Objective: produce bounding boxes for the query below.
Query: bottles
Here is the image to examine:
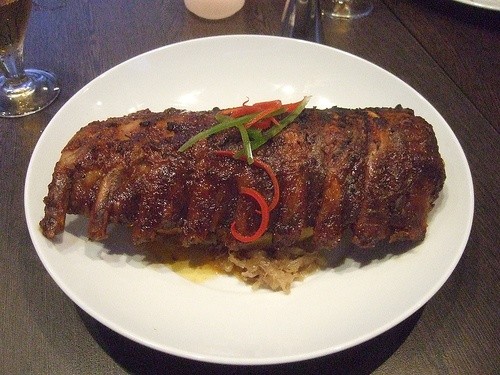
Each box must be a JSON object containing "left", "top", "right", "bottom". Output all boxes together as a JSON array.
[{"left": 280, "top": 0, "right": 322, "bottom": 45}]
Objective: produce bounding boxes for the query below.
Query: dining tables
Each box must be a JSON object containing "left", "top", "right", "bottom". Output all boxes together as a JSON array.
[{"left": 0, "top": 0, "right": 500, "bottom": 375}]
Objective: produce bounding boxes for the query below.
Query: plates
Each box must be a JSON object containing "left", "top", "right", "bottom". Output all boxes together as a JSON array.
[{"left": 23, "top": 34, "right": 475, "bottom": 366}]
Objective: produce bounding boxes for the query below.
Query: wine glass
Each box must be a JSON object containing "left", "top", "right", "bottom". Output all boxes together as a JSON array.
[
  {"left": 321, "top": 0, "right": 375, "bottom": 19},
  {"left": 0, "top": 0, "right": 61, "bottom": 117}
]
